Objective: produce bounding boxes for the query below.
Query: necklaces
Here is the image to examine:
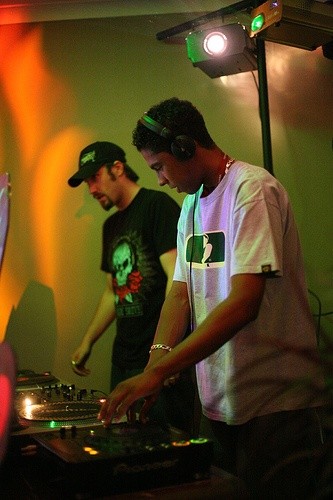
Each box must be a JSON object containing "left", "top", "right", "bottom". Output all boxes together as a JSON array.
[{"left": 219, "top": 153, "right": 234, "bottom": 183}]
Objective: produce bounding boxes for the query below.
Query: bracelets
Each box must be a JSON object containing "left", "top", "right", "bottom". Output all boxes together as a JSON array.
[{"left": 149, "top": 344, "right": 173, "bottom": 354}]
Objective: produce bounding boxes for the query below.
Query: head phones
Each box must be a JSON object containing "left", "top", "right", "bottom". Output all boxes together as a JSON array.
[{"left": 139, "top": 112, "right": 196, "bottom": 161}]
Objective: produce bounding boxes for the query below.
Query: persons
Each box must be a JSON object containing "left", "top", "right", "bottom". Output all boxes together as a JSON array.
[
  {"left": 97, "top": 97, "right": 328, "bottom": 477},
  {"left": 68, "top": 141, "right": 196, "bottom": 437}
]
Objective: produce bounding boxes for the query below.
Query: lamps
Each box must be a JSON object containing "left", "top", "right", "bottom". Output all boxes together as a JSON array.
[{"left": 185, "top": 23, "right": 258, "bottom": 79}]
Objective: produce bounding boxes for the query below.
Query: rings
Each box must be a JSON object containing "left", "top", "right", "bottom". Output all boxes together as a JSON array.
[{"left": 71, "top": 360, "right": 76, "bottom": 365}]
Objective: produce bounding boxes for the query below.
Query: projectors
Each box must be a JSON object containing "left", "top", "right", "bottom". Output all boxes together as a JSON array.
[{"left": 185, "top": 23, "right": 259, "bottom": 79}]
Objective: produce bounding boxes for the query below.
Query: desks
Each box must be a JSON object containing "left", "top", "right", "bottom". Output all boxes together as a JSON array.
[{"left": 21, "top": 464, "right": 243, "bottom": 500}]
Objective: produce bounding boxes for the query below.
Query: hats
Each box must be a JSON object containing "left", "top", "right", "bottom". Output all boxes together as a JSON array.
[{"left": 68, "top": 141, "right": 126, "bottom": 188}]
